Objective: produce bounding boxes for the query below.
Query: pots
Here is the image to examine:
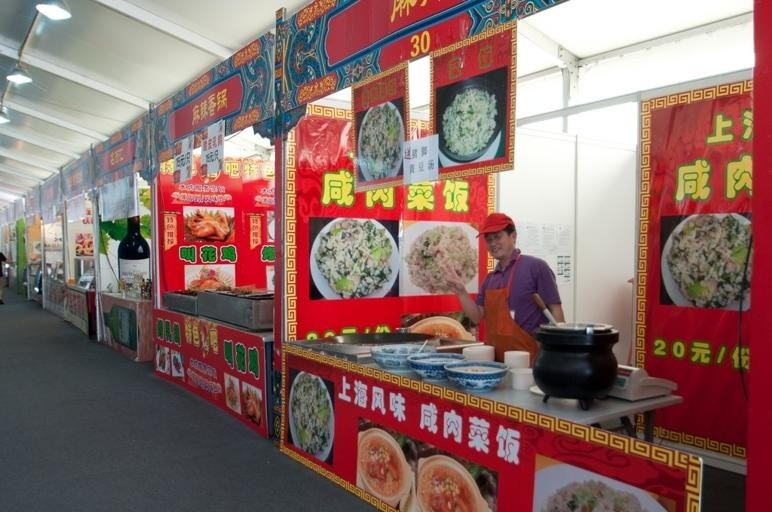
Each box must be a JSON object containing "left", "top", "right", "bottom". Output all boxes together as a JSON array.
[
  {"left": 533, "top": 323, "right": 618, "bottom": 410},
  {"left": 357, "top": 428, "right": 414, "bottom": 503},
  {"left": 413, "top": 454, "right": 489, "bottom": 512},
  {"left": 438, "top": 78, "right": 503, "bottom": 160}
]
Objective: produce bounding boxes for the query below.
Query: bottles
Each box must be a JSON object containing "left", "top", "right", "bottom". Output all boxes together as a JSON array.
[{"left": 115, "top": 216, "right": 149, "bottom": 293}]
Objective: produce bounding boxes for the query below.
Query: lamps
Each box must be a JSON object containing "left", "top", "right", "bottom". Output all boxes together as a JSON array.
[
  {"left": 35, "top": 1, "right": 72, "bottom": 20},
  {"left": 6, "top": 60, "right": 32, "bottom": 83},
  {"left": 0, "top": 108, "right": 9, "bottom": 120}
]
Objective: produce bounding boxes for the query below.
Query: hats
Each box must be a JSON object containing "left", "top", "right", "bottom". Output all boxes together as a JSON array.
[{"left": 475, "top": 215, "right": 517, "bottom": 236}]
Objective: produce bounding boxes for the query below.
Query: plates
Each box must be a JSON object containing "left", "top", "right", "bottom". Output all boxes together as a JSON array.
[
  {"left": 156, "top": 350, "right": 170, "bottom": 373},
  {"left": 357, "top": 102, "right": 405, "bottom": 181},
  {"left": 533, "top": 463, "right": 669, "bottom": 511},
  {"left": 242, "top": 381, "right": 261, "bottom": 426},
  {"left": 172, "top": 353, "right": 186, "bottom": 379},
  {"left": 224, "top": 373, "right": 241, "bottom": 415}
]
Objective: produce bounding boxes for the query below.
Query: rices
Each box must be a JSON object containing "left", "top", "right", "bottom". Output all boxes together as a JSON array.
[
  {"left": 361, "top": 104, "right": 402, "bottom": 178},
  {"left": 441, "top": 86, "right": 499, "bottom": 156},
  {"left": 542, "top": 475, "right": 645, "bottom": 512}
]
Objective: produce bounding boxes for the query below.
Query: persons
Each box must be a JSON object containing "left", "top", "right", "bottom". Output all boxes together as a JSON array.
[
  {"left": 1, "top": 251, "right": 8, "bottom": 303},
  {"left": 446, "top": 214, "right": 565, "bottom": 368}
]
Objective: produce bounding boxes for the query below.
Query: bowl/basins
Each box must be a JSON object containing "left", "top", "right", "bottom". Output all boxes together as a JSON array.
[
  {"left": 444, "top": 359, "right": 508, "bottom": 393},
  {"left": 370, "top": 343, "right": 436, "bottom": 374},
  {"left": 407, "top": 316, "right": 473, "bottom": 342},
  {"left": 408, "top": 353, "right": 464, "bottom": 383}
]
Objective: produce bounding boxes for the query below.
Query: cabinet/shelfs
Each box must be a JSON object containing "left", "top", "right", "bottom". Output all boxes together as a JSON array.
[
  {"left": 159, "top": 307, "right": 274, "bottom": 439},
  {"left": 68, "top": 284, "right": 96, "bottom": 339},
  {"left": 45, "top": 277, "right": 65, "bottom": 318},
  {"left": 285, "top": 343, "right": 682, "bottom": 512},
  {"left": 100, "top": 293, "right": 153, "bottom": 362}
]
